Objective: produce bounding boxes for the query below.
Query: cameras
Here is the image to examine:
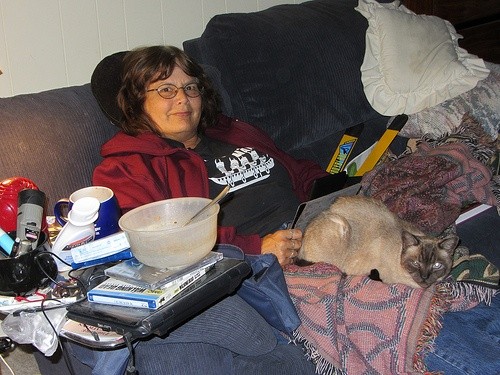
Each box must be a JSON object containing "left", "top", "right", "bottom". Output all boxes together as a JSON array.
[{"left": 0, "top": 254, "right": 57, "bottom": 296}]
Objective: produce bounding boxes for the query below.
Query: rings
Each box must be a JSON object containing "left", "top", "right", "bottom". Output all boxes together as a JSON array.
[
  {"left": 288, "top": 229, "right": 293, "bottom": 240},
  {"left": 288, "top": 249, "right": 294, "bottom": 259},
  {"left": 290, "top": 240, "right": 295, "bottom": 249}
]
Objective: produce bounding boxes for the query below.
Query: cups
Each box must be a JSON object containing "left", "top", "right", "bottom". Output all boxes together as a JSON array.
[
  {"left": 0, "top": 229, "right": 58, "bottom": 297},
  {"left": 54, "top": 186, "right": 123, "bottom": 241}
]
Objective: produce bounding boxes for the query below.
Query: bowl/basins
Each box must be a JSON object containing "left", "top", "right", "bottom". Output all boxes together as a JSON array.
[{"left": 120, "top": 194, "right": 221, "bottom": 269}]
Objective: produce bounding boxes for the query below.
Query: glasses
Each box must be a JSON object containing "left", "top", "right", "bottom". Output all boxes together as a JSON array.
[{"left": 144, "top": 82, "right": 204, "bottom": 99}]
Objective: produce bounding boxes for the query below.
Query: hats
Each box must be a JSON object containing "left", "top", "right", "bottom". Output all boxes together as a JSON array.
[{"left": 91, "top": 51, "right": 139, "bottom": 127}]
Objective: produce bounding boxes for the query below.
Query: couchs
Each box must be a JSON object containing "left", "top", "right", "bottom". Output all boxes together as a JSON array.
[{"left": 0, "top": 0, "right": 500, "bottom": 375}]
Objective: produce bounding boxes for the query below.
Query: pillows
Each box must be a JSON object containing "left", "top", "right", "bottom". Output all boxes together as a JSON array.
[{"left": 351, "top": 0, "right": 490, "bottom": 116}]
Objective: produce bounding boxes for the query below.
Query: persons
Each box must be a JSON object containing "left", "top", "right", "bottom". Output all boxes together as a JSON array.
[{"left": 93, "top": 44, "right": 500, "bottom": 375}]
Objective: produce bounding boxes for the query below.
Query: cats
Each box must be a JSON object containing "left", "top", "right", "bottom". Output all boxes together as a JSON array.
[{"left": 290, "top": 194, "right": 460, "bottom": 290}]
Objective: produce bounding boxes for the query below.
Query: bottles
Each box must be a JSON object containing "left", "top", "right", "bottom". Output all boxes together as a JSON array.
[{"left": 50, "top": 197, "right": 99, "bottom": 272}]
